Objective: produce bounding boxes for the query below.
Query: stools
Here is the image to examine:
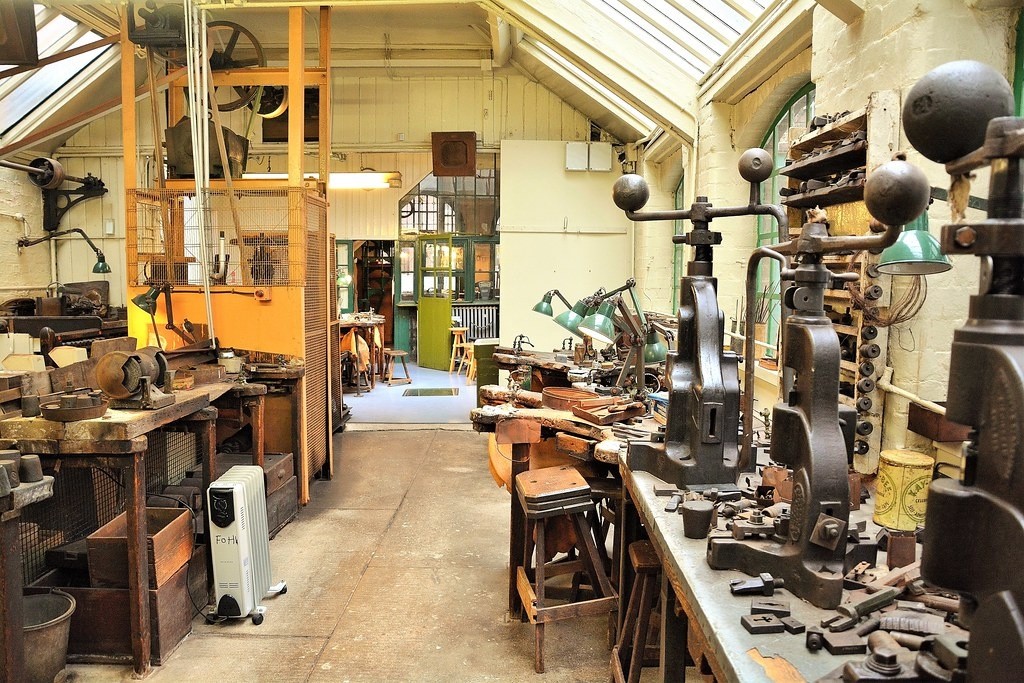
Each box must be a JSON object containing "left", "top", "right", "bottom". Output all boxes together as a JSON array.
[
  {"left": 516, "top": 465, "right": 619, "bottom": 673},
  {"left": 607, "top": 541, "right": 660, "bottom": 683},
  {"left": 449, "top": 327, "right": 477, "bottom": 386},
  {"left": 341, "top": 348, "right": 412, "bottom": 393}
]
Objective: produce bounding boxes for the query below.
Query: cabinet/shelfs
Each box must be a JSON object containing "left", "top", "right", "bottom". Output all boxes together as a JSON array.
[{"left": 362, "top": 241, "right": 395, "bottom": 345}]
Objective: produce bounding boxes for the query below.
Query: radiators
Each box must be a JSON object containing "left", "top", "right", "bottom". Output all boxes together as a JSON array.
[
  {"left": 452, "top": 307, "right": 498, "bottom": 342},
  {"left": 205, "top": 465, "right": 288, "bottom": 623}
]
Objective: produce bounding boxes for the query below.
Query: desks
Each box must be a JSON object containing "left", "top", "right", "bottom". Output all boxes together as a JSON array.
[
  {"left": 339, "top": 315, "right": 387, "bottom": 387},
  {"left": 618, "top": 444, "right": 921, "bottom": 683}
]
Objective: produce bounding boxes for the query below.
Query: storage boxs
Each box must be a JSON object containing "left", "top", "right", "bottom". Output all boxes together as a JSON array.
[{"left": 26, "top": 452, "right": 300, "bottom": 665}]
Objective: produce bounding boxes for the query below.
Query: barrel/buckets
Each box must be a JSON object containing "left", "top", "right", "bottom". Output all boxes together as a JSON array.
[{"left": 22, "top": 587, "right": 76, "bottom": 683}]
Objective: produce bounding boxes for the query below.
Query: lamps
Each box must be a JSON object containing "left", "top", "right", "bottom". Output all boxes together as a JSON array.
[
  {"left": 531, "top": 278, "right": 670, "bottom": 393},
  {"left": 241, "top": 172, "right": 402, "bottom": 187},
  {"left": 336, "top": 268, "right": 352, "bottom": 286},
  {"left": 19, "top": 230, "right": 112, "bottom": 273},
  {"left": 876, "top": 187, "right": 988, "bottom": 276},
  {"left": 130, "top": 281, "right": 193, "bottom": 345}
]
